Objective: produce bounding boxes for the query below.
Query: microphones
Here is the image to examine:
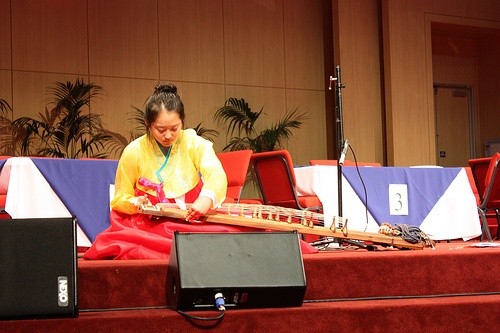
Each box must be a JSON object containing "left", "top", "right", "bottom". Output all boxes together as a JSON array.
[{"left": 339, "top": 140, "right": 348, "bottom": 166}]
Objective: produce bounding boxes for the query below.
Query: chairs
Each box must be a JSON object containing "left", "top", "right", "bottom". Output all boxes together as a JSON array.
[{"left": 220, "top": 149, "right": 500, "bottom": 244}]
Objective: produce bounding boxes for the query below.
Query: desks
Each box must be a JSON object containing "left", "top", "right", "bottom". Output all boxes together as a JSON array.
[
  {"left": 2, "top": 158, "right": 119, "bottom": 248},
  {"left": 293, "top": 164, "right": 483, "bottom": 243}
]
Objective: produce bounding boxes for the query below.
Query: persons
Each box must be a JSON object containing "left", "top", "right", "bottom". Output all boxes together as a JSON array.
[{"left": 82, "top": 84, "right": 320, "bottom": 260}]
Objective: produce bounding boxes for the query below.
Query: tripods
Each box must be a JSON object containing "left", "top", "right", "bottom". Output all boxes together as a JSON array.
[{"left": 306, "top": 66, "right": 374, "bottom": 251}]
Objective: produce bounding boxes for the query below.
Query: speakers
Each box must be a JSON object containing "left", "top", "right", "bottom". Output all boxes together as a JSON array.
[
  {"left": 0, "top": 216, "right": 78, "bottom": 320},
  {"left": 166, "top": 230, "right": 307, "bottom": 311}
]
